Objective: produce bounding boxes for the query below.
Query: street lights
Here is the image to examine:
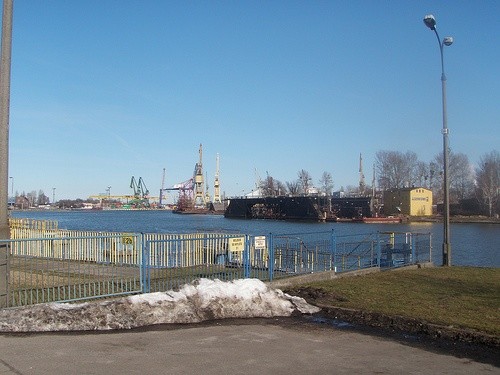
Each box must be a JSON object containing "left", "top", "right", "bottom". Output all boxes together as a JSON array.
[
  {"left": 9, "top": 176, "right": 14, "bottom": 207},
  {"left": 423, "top": 12, "right": 455, "bottom": 266}
]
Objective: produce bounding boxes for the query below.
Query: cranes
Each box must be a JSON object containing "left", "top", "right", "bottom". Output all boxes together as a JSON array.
[
  {"left": 358, "top": 153, "right": 366, "bottom": 194},
  {"left": 214, "top": 151, "right": 222, "bottom": 204},
  {"left": 194, "top": 142, "right": 207, "bottom": 208},
  {"left": 161, "top": 166, "right": 170, "bottom": 200},
  {"left": 205, "top": 171, "right": 210, "bottom": 204}
]
